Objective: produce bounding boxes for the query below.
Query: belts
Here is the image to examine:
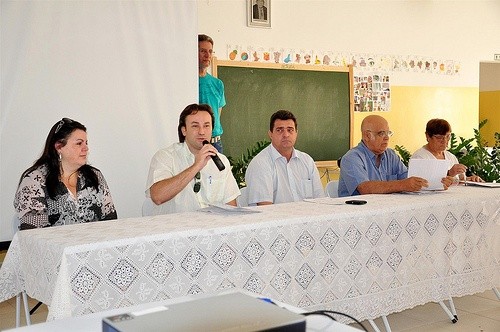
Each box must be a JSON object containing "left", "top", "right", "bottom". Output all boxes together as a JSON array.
[{"left": 210, "top": 134, "right": 223, "bottom": 144}]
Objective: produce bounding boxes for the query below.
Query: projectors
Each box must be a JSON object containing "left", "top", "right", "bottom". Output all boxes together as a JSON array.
[{"left": 102, "top": 291, "right": 307, "bottom": 332}]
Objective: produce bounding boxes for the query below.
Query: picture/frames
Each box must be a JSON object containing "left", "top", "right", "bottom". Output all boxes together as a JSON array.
[{"left": 246, "top": 0, "right": 272, "bottom": 29}]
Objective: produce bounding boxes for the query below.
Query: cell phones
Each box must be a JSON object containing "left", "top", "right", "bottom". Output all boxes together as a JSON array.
[{"left": 345, "top": 199, "right": 367, "bottom": 205}]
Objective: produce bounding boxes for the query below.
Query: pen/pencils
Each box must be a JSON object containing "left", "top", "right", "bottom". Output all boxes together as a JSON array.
[{"left": 463, "top": 170, "right": 467, "bottom": 187}]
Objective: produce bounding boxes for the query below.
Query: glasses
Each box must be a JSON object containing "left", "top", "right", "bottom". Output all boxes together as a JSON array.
[
  {"left": 198, "top": 48, "right": 215, "bottom": 56},
  {"left": 194, "top": 172, "right": 200, "bottom": 193},
  {"left": 365, "top": 129, "right": 393, "bottom": 138},
  {"left": 434, "top": 134, "right": 453, "bottom": 141},
  {"left": 54, "top": 118, "right": 75, "bottom": 134}
]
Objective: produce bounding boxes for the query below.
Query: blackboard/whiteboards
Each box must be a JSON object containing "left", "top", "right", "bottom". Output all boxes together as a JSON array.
[{"left": 212, "top": 57, "right": 353, "bottom": 166}]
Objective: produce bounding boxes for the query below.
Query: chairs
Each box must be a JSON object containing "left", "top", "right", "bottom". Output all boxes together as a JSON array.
[{"left": 325, "top": 180, "right": 339, "bottom": 197}]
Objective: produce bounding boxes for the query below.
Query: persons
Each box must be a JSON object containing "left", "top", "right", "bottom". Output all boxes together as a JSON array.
[
  {"left": 253, "top": 0, "right": 267, "bottom": 20},
  {"left": 338, "top": 115, "right": 454, "bottom": 197},
  {"left": 198, "top": 34, "right": 226, "bottom": 153},
  {"left": 245, "top": 110, "right": 323, "bottom": 206},
  {"left": 412, "top": 118, "right": 482, "bottom": 183},
  {"left": 145, "top": 104, "right": 241, "bottom": 215},
  {"left": 13, "top": 118, "right": 117, "bottom": 230}
]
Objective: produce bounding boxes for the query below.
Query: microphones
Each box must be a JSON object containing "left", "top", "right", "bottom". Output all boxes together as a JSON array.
[{"left": 202, "top": 139, "right": 225, "bottom": 171}]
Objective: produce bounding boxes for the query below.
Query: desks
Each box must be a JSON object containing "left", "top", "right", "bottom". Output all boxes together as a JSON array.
[{"left": 0, "top": 185, "right": 500, "bottom": 332}]
